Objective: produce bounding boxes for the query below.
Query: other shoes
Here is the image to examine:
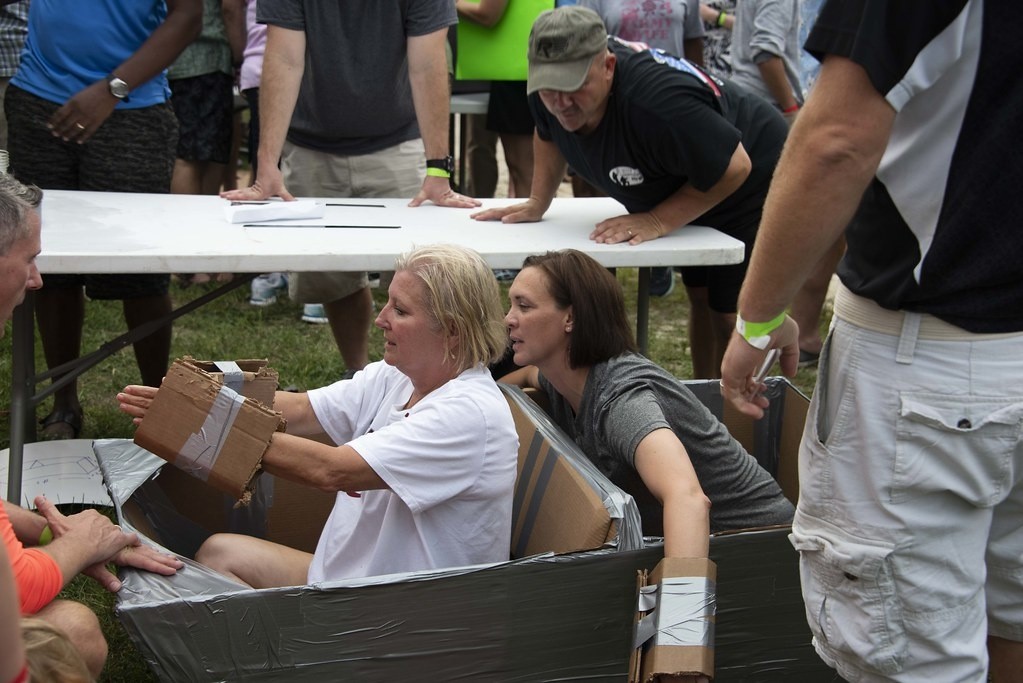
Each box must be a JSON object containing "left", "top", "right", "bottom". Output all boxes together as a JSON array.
[
  {"left": 301, "top": 299, "right": 375, "bottom": 323},
  {"left": 650, "top": 266, "right": 675, "bottom": 297},
  {"left": 250, "top": 278, "right": 280, "bottom": 305},
  {"left": 493, "top": 270, "right": 521, "bottom": 283}
]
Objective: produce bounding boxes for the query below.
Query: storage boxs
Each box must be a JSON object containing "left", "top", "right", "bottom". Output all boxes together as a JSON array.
[{"left": 87, "top": 376, "right": 847, "bottom": 683}]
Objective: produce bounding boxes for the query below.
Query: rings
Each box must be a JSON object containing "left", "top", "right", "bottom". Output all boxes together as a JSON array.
[
  {"left": 115, "top": 525, "right": 122, "bottom": 532},
  {"left": 77, "top": 123, "right": 85, "bottom": 130},
  {"left": 628, "top": 231, "right": 632, "bottom": 235}
]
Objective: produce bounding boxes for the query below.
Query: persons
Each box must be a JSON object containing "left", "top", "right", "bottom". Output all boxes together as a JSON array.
[
  {"left": 0, "top": 171, "right": 183, "bottom": 683},
  {"left": 469, "top": 7, "right": 784, "bottom": 381},
  {"left": 116, "top": 242, "right": 519, "bottom": 591},
  {"left": 577, "top": 0, "right": 735, "bottom": 300},
  {"left": 502, "top": 250, "right": 797, "bottom": 681},
  {"left": 455, "top": 0, "right": 554, "bottom": 194},
  {"left": 0, "top": 0, "right": 266, "bottom": 437},
  {"left": 719, "top": 0, "right": 1023, "bottom": 683},
  {"left": 219, "top": 0, "right": 478, "bottom": 378},
  {"left": 732, "top": 0, "right": 846, "bottom": 361}
]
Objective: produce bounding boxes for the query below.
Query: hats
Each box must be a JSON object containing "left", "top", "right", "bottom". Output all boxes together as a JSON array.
[{"left": 526, "top": 4, "right": 608, "bottom": 94}]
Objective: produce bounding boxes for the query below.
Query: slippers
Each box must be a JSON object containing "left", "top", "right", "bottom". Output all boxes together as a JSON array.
[
  {"left": 42, "top": 407, "right": 86, "bottom": 441},
  {"left": 797, "top": 348, "right": 819, "bottom": 367}
]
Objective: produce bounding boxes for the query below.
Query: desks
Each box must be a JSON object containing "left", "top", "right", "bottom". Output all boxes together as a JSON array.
[{"left": 8, "top": 188, "right": 744, "bottom": 506}]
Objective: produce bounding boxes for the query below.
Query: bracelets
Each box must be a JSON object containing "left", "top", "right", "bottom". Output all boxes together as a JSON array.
[
  {"left": 719, "top": 12, "right": 726, "bottom": 25},
  {"left": 734, "top": 313, "right": 785, "bottom": 349},
  {"left": 785, "top": 106, "right": 798, "bottom": 112},
  {"left": 717, "top": 11, "right": 722, "bottom": 24},
  {"left": 427, "top": 168, "right": 450, "bottom": 177}
]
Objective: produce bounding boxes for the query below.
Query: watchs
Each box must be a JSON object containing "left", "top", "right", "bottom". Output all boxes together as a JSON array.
[
  {"left": 427, "top": 156, "right": 453, "bottom": 171},
  {"left": 106, "top": 75, "right": 129, "bottom": 103}
]
